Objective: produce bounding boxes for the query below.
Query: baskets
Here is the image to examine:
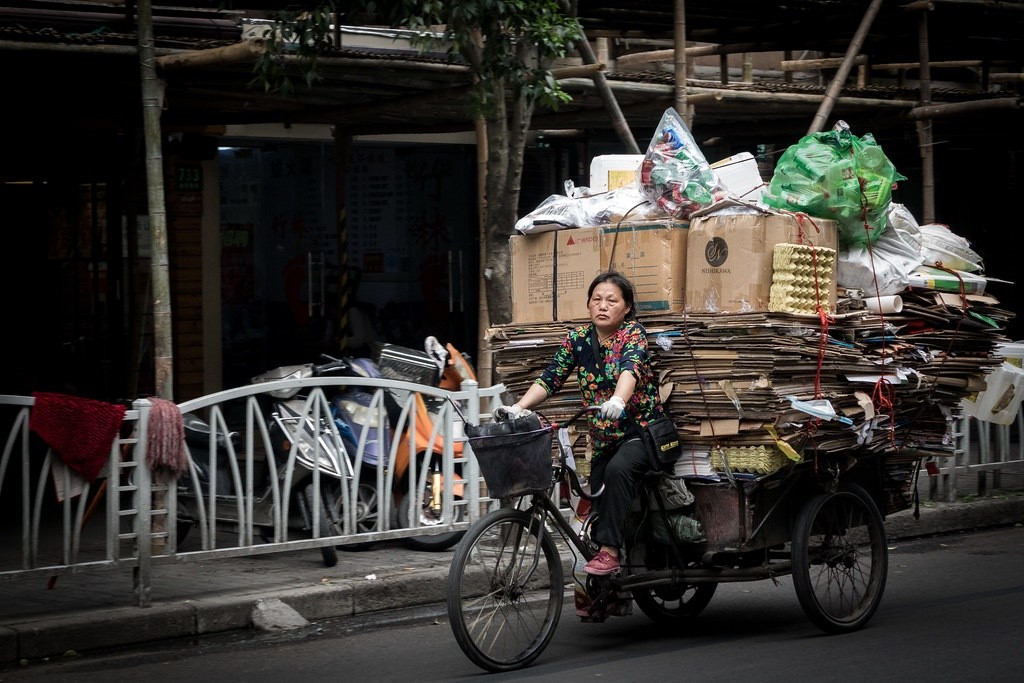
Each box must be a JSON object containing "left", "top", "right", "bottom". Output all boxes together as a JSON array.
[{"left": 468, "top": 427, "right": 552, "bottom": 498}]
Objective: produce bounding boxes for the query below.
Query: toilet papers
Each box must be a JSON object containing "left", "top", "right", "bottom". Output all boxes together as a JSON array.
[{"left": 863, "top": 295, "right": 903, "bottom": 314}]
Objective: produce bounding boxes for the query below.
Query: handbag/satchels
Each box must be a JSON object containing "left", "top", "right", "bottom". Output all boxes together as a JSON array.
[
  {"left": 641, "top": 417, "right": 682, "bottom": 470},
  {"left": 463, "top": 412, "right": 543, "bottom": 447}
]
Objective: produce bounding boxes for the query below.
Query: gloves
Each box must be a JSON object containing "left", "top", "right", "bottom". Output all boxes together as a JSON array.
[
  {"left": 600, "top": 396, "right": 626, "bottom": 420},
  {"left": 492, "top": 404, "right": 522, "bottom": 423}
]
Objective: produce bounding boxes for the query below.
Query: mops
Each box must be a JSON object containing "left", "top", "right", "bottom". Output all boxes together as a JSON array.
[{"left": 46, "top": 396, "right": 189, "bottom": 591}]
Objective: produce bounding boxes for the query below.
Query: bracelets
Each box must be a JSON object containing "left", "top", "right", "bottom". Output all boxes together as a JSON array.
[{"left": 516, "top": 402, "right": 524, "bottom": 410}]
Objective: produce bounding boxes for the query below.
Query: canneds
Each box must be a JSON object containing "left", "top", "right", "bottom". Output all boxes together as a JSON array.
[{"left": 641, "top": 125, "right": 725, "bottom": 218}]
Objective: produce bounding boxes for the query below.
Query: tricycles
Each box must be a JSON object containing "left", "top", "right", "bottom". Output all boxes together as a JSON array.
[{"left": 445, "top": 405, "right": 916, "bottom": 673}]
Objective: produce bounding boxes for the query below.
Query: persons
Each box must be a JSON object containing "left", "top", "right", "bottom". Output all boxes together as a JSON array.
[{"left": 492, "top": 272, "right": 665, "bottom": 616}]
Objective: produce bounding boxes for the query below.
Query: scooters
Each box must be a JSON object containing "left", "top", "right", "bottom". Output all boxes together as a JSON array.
[{"left": 109, "top": 337, "right": 478, "bottom": 568}]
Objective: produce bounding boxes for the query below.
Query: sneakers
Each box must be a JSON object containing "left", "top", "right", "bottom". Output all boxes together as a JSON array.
[
  {"left": 576, "top": 599, "right": 616, "bottom": 616},
  {"left": 583, "top": 551, "right": 620, "bottom": 575}
]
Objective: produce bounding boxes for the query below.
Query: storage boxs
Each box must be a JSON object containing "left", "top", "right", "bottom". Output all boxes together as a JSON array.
[
  {"left": 508, "top": 154, "right": 840, "bottom": 329},
  {"left": 959, "top": 342, "right": 1024, "bottom": 426},
  {"left": 375, "top": 343, "right": 438, "bottom": 393}
]
